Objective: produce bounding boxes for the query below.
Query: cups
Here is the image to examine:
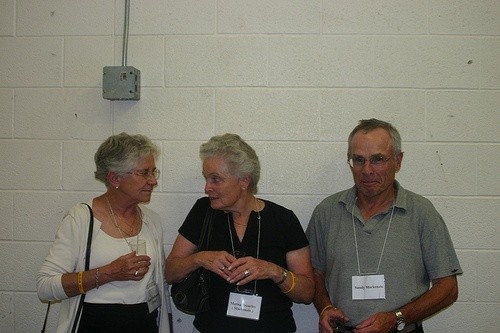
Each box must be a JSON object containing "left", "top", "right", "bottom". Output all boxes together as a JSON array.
[
  {"left": 128, "top": 238, "right": 146, "bottom": 270},
  {"left": 329, "top": 310, "right": 345, "bottom": 333}
]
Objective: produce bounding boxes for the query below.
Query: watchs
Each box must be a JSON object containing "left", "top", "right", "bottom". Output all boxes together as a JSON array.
[{"left": 392, "top": 308, "right": 405, "bottom": 331}]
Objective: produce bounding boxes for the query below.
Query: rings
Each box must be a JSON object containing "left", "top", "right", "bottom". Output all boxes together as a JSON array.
[
  {"left": 245, "top": 270, "right": 250, "bottom": 276},
  {"left": 221, "top": 267, "right": 225, "bottom": 270},
  {"left": 135, "top": 271, "right": 138, "bottom": 276}
]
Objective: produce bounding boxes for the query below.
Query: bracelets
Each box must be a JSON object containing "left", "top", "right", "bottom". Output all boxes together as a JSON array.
[
  {"left": 275, "top": 269, "right": 288, "bottom": 285},
  {"left": 320, "top": 305, "right": 336, "bottom": 316},
  {"left": 282, "top": 271, "right": 296, "bottom": 293},
  {"left": 96, "top": 267, "right": 99, "bottom": 289},
  {"left": 78, "top": 271, "right": 86, "bottom": 294}
]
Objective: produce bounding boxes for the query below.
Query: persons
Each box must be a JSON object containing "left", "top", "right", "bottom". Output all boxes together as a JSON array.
[
  {"left": 305, "top": 117, "right": 463, "bottom": 333},
  {"left": 164, "top": 133, "right": 316, "bottom": 333},
  {"left": 36, "top": 131, "right": 174, "bottom": 333}
]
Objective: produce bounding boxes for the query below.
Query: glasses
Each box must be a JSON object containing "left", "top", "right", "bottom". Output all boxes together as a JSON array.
[
  {"left": 126, "top": 169, "right": 161, "bottom": 180},
  {"left": 347, "top": 155, "right": 394, "bottom": 169}
]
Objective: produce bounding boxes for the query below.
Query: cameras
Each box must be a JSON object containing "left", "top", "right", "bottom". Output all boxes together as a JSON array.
[{"left": 331, "top": 318, "right": 356, "bottom": 333}]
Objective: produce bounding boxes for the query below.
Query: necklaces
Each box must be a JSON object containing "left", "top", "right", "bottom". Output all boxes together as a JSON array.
[{"left": 105, "top": 195, "right": 137, "bottom": 231}]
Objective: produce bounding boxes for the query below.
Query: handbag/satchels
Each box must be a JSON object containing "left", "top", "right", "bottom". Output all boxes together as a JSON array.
[{"left": 171, "top": 269, "right": 210, "bottom": 315}]
